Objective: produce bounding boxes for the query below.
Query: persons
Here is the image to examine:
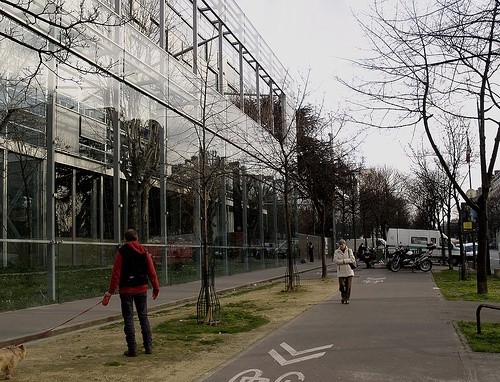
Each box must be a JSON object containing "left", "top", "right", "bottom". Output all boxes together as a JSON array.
[
  {"left": 334, "top": 239, "right": 356, "bottom": 303},
  {"left": 103, "top": 229, "right": 160, "bottom": 357},
  {"left": 358, "top": 243, "right": 367, "bottom": 261},
  {"left": 308, "top": 242, "right": 314, "bottom": 262}
]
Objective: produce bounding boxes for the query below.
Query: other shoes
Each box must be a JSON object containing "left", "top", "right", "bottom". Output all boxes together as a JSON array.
[
  {"left": 341, "top": 298, "right": 345, "bottom": 304},
  {"left": 145, "top": 347, "right": 152, "bottom": 354},
  {"left": 124, "top": 350, "right": 137, "bottom": 357},
  {"left": 346, "top": 300, "right": 349, "bottom": 303}
]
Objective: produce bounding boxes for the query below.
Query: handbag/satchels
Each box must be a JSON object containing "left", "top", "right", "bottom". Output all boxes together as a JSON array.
[{"left": 348, "top": 249, "right": 358, "bottom": 270}]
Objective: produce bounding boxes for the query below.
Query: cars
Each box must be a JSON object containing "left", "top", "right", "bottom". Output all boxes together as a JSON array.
[
  {"left": 254, "top": 239, "right": 300, "bottom": 259},
  {"left": 463, "top": 242, "right": 478, "bottom": 261}
]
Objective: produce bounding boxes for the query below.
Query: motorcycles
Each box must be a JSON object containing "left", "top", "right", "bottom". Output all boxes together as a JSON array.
[{"left": 360, "top": 241, "right": 437, "bottom": 273}]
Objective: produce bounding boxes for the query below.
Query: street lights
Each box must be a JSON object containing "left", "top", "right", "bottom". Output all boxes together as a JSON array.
[{"left": 466, "top": 189, "right": 477, "bottom": 269}]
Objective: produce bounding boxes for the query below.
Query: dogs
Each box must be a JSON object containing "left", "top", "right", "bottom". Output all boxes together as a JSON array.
[{"left": 0, "top": 343, "right": 29, "bottom": 380}]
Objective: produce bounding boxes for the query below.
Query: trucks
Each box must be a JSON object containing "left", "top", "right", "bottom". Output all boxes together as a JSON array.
[
  {"left": 385, "top": 228, "right": 460, "bottom": 267},
  {"left": 345, "top": 237, "right": 386, "bottom": 259}
]
